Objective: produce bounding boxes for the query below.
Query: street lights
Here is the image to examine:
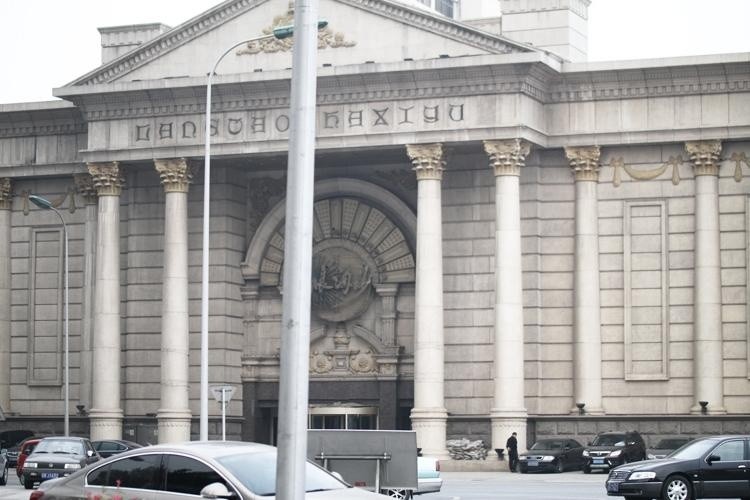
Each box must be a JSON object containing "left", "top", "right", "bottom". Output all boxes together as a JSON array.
[
  {"left": 199, "top": 20, "right": 328, "bottom": 440},
  {"left": 29, "top": 193, "right": 70, "bottom": 438}
]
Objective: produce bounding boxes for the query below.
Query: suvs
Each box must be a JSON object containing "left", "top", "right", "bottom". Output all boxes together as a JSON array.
[{"left": 581, "top": 430, "right": 647, "bottom": 473}]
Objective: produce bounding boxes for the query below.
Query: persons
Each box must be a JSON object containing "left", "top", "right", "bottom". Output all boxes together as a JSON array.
[{"left": 506, "top": 431, "right": 519, "bottom": 473}]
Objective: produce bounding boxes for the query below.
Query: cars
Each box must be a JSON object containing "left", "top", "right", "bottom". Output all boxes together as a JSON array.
[
  {"left": 605, "top": 435, "right": 750, "bottom": 500},
  {"left": 30, "top": 442, "right": 398, "bottom": 500},
  {"left": 645, "top": 437, "right": 697, "bottom": 460},
  {"left": 381, "top": 458, "right": 442, "bottom": 500},
  {"left": 517, "top": 438, "right": 589, "bottom": 473},
  {"left": 0, "top": 437, "right": 147, "bottom": 489}
]
